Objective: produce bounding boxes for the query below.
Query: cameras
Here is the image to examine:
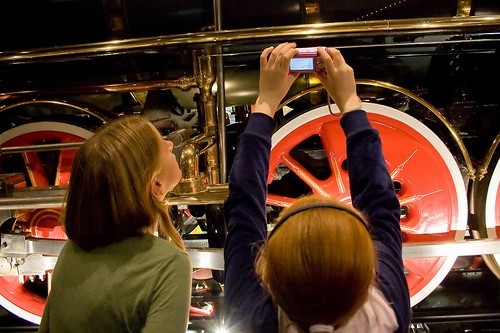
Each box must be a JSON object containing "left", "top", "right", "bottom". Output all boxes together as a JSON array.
[{"left": 288, "top": 47, "right": 327, "bottom": 74}]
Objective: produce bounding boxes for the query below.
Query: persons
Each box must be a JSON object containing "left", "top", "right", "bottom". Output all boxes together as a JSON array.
[
  {"left": 221, "top": 42, "right": 413, "bottom": 333},
  {"left": 37, "top": 114, "right": 193, "bottom": 333}
]
